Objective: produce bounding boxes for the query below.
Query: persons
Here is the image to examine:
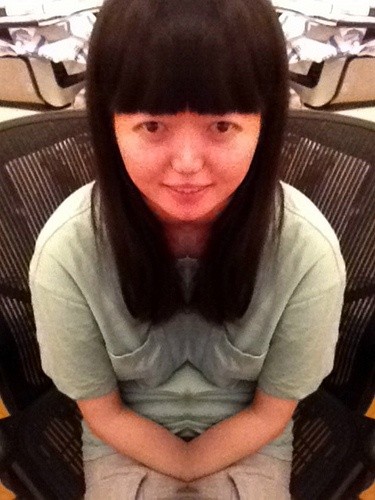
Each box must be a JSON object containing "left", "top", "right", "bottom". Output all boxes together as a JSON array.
[{"left": 25, "top": 0, "right": 347, "bottom": 500}]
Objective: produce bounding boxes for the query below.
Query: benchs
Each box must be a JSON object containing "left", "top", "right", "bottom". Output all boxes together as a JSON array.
[{"left": 1, "top": 0, "right": 375, "bottom": 110}]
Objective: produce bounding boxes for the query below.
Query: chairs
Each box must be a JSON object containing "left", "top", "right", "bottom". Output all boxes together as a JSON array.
[{"left": 1, "top": 102, "right": 375, "bottom": 500}]
